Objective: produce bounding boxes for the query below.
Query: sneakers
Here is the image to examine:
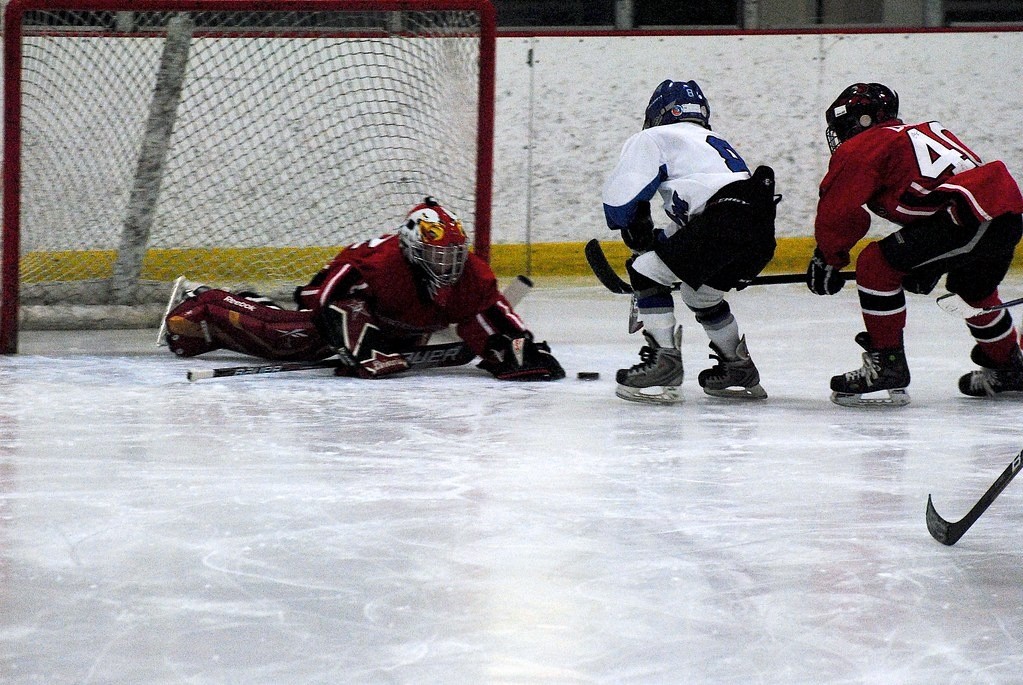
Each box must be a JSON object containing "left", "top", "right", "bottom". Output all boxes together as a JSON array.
[
  {"left": 614, "top": 325, "right": 686, "bottom": 403},
  {"left": 830, "top": 332, "right": 911, "bottom": 409},
  {"left": 958, "top": 344, "right": 1023, "bottom": 397},
  {"left": 698, "top": 334, "right": 768, "bottom": 399}
]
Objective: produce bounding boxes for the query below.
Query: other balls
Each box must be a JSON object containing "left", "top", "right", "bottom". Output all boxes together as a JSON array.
[{"left": 577, "top": 371, "right": 600, "bottom": 381}]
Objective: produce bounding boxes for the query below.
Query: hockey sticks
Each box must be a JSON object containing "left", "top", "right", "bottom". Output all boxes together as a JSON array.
[
  {"left": 924, "top": 448, "right": 1023, "bottom": 548},
  {"left": 583, "top": 238, "right": 857, "bottom": 296},
  {"left": 185, "top": 271, "right": 536, "bottom": 382},
  {"left": 628, "top": 273, "right": 645, "bottom": 336}
]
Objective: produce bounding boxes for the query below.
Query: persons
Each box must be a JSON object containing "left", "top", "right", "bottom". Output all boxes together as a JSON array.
[
  {"left": 600, "top": 78, "right": 778, "bottom": 404},
  {"left": 807, "top": 82, "right": 1023, "bottom": 408},
  {"left": 165, "top": 203, "right": 565, "bottom": 382}
]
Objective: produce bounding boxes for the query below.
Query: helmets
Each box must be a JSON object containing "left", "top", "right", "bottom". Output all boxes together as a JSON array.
[
  {"left": 826, "top": 83, "right": 899, "bottom": 155},
  {"left": 642, "top": 79, "right": 712, "bottom": 131},
  {"left": 398, "top": 196, "right": 468, "bottom": 288}
]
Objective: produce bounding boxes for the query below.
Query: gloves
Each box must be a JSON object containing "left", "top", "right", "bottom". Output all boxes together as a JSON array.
[
  {"left": 619, "top": 203, "right": 654, "bottom": 251},
  {"left": 902, "top": 275, "right": 944, "bottom": 295},
  {"left": 805, "top": 248, "right": 847, "bottom": 295}
]
